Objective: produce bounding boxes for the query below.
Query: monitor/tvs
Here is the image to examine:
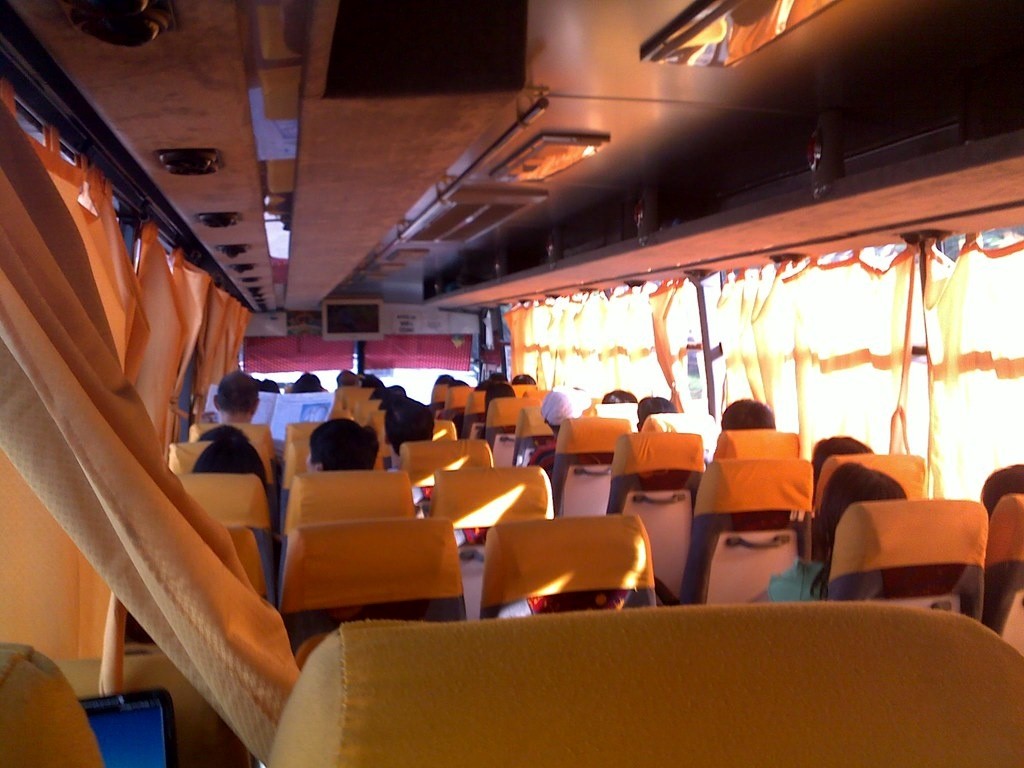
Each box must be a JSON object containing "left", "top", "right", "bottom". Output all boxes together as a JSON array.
[{"left": 321, "top": 295, "right": 383, "bottom": 341}]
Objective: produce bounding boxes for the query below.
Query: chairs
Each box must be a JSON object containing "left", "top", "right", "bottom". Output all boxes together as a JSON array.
[{"left": 1, "top": 384, "right": 1024, "bottom": 768}]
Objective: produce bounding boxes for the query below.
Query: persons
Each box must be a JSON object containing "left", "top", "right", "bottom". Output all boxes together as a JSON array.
[
  {"left": 524, "top": 390, "right": 584, "bottom": 485},
  {"left": 452, "top": 380, "right": 494, "bottom": 438},
  {"left": 191, "top": 439, "right": 267, "bottom": 485},
  {"left": 811, "top": 436, "right": 874, "bottom": 499},
  {"left": 513, "top": 375, "right": 538, "bottom": 385},
  {"left": 434, "top": 375, "right": 469, "bottom": 386},
  {"left": 305, "top": 419, "right": 379, "bottom": 472},
  {"left": 603, "top": 390, "right": 637, "bottom": 403},
  {"left": 635, "top": 398, "right": 679, "bottom": 432},
  {"left": 476, "top": 383, "right": 516, "bottom": 438},
  {"left": 976, "top": 465, "right": 1024, "bottom": 519},
  {"left": 489, "top": 372, "right": 509, "bottom": 382},
  {"left": 761, "top": 462, "right": 907, "bottom": 602},
  {"left": 196, "top": 425, "right": 249, "bottom": 441},
  {"left": 213, "top": 369, "right": 280, "bottom": 425},
  {"left": 336, "top": 370, "right": 435, "bottom": 471},
  {"left": 291, "top": 373, "right": 321, "bottom": 393},
  {"left": 720, "top": 399, "right": 776, "bottom": 431}
]
